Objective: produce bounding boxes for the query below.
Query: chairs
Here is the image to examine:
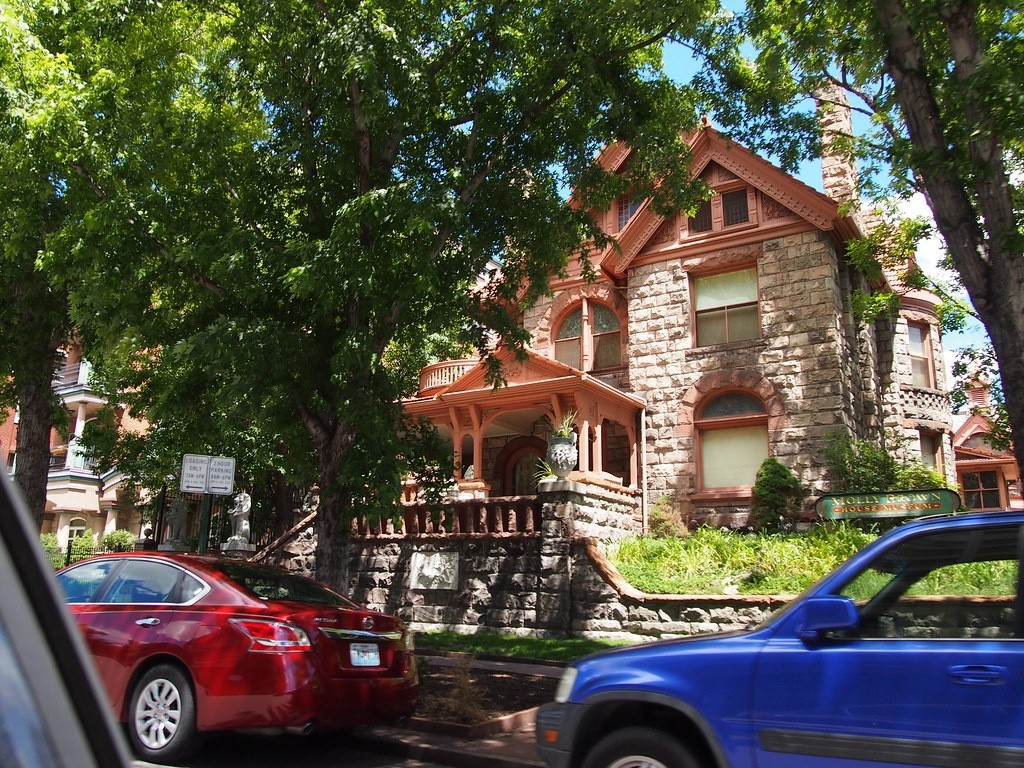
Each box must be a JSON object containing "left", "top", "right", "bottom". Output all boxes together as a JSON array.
[{"left": 181, "top": 575, "right": 202, "bottom": 601}]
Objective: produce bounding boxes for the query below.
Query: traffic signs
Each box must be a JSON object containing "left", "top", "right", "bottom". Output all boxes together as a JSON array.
[{"left": 180, "top": 454, "right": 236, "bottom": 497}]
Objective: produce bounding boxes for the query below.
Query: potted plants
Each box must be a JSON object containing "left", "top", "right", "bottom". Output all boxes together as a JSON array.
[
  {"left": 101, "top": 528, "right": 139, "bottom": 553},
  {"left": 751, "top": 458, "right": 803, "bottom": 533},
  {"left": 542, "top": 403, "right": 584, "bottom": 480}
]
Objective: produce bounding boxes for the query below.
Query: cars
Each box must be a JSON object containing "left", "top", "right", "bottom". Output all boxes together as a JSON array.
[
  {"left": 533, "top": 511, "right": 1024, "bottom": 768},
  {"left": 44, "top": 548, "right": 424, "bottom": 763}
]
